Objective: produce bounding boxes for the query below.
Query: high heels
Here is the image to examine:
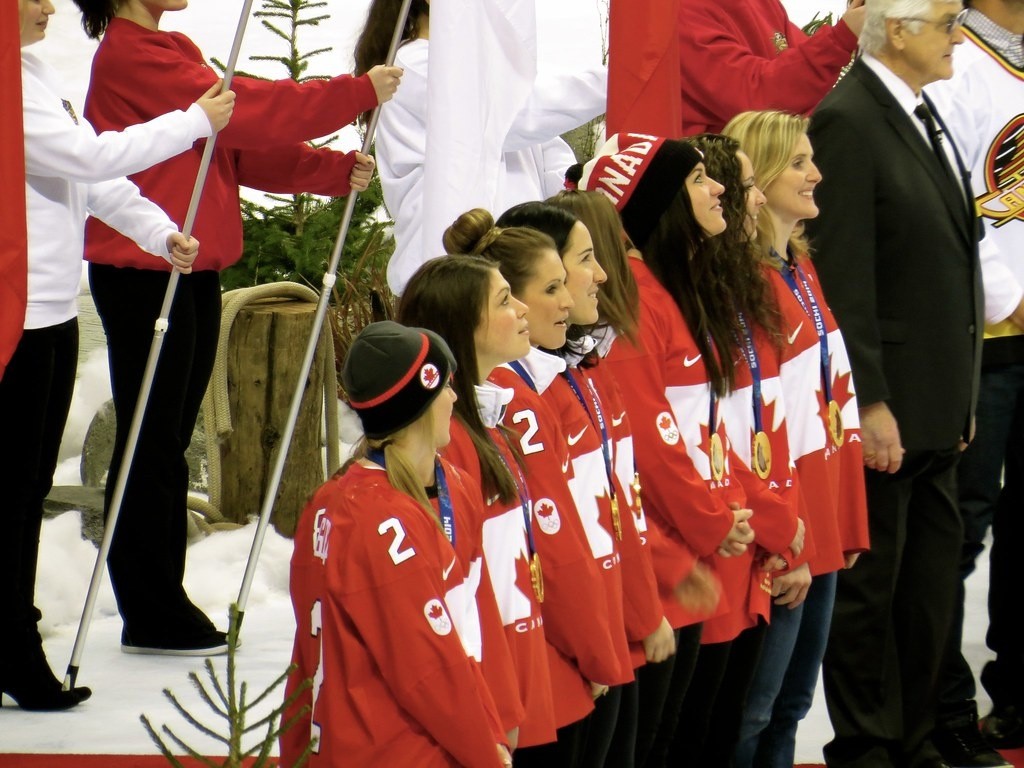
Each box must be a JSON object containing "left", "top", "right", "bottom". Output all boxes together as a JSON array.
[{"left": 0, "top": 621, "right": 91, "bottom": 713}]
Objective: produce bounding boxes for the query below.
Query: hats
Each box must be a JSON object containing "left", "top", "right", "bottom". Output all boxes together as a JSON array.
[
  {"left": 564, "top": 129, "right": 666, "bottom": 212},
  {"left": 342, "top": 321, "right": 459, "bottom": 441}
]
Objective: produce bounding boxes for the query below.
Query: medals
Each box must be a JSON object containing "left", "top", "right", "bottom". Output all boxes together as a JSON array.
[
  {"left": 753, "top": 430, "right": 771, "bottom": 479},
  {"left": 529, "top": 550, "right": 545, "bottom": 601},
  {"left": 827, "top": 399, "right": 844, "bottom": 447},
  {"left": 611, "top": 490, "right": 624, "bottom": 541},
  {"left": 709, "top": 431, "right": 724, "bottom": 481},
  {"left": 632, "top": 470, "right": 645, "bottom": 517}
]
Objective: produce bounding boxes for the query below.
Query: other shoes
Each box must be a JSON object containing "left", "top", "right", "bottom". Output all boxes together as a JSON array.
[
  {"left": 930, "top": 698, "right": 1004, "bottom": 767},
  {"left": 983, "top": 711, "right": 1024, "bottom": 747},
  {"left": 120, "top": 598, "right": 242, "bottom": 656}
]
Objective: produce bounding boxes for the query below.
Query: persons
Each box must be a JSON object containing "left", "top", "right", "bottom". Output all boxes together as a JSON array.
[
  {"left": 73, "top": 0, "right": 404, "bottom": 658},
  {"left": 677, "top": 0, "right": 866, "bottom": 135},
  {"left": 1, "top": 0, "right": 235, "bottom": 712},
  {"left": 354, "top": 0, "right": 580, "bottom": 300},
  {"left": 924, "top": 1, "right": 1023, "bottom": 767},
  {"left": 399, "top": 112, "right": 869, "bottom": 768},
  {"left": 276, "top": 322, "right": 524, "bottom": 767},
  {"left": 810, "top": 1, "right": 1018, "bottom": 767}
]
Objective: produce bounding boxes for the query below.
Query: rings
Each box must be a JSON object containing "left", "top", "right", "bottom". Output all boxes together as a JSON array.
[{"left": 865, "top": 451, "right": 875, "bottom": 461}]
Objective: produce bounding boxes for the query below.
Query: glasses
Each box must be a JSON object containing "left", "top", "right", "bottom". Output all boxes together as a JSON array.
[{"left": 895, "top": 7, "right": 970, "bottom": 33}]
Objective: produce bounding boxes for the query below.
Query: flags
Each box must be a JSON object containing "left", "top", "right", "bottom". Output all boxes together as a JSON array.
[
  {"left": 0, "top": 0, "right": 28, "bottom": 367},
  {"left": 416, "top": 0, "right": 683, "bottom": 270}
]
